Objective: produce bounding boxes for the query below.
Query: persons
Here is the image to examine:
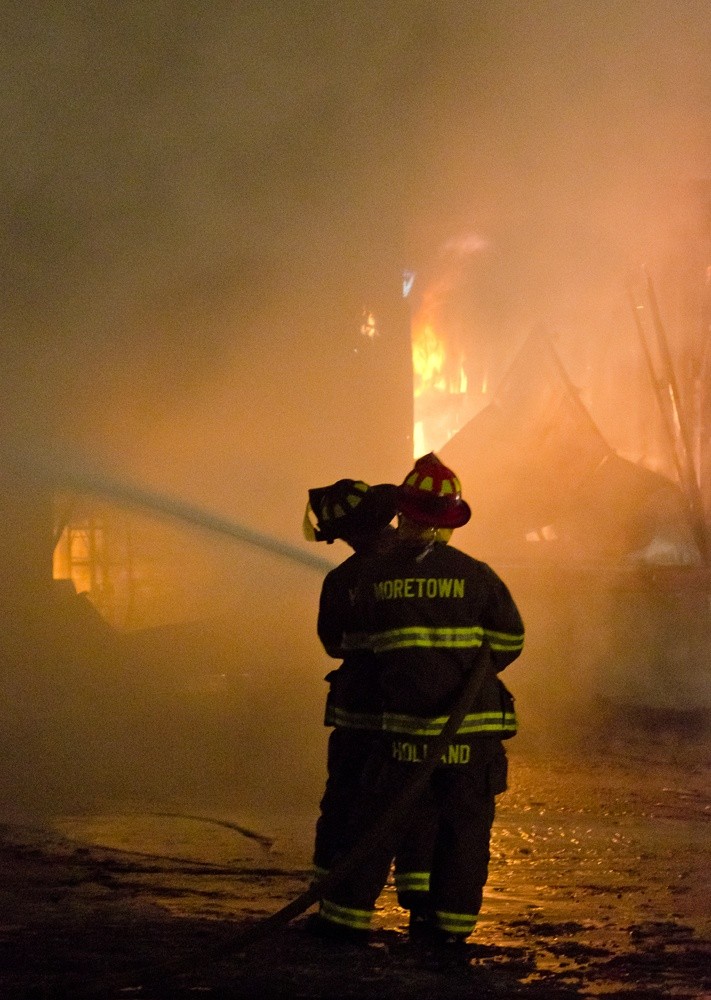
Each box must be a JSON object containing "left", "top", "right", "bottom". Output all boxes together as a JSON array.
[
  {"left": 304, "top": 479, "right": 439, "bottom": 935},
  {"left": 305, "top": 452, "right": 525, "bottom": 962}
]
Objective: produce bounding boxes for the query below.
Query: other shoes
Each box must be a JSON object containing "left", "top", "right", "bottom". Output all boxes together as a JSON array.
[
  {"left": 409, "top": 928, "right": 467, "bottom": 968},
  {"left": 307, "top": 911, "right": 369, "bottom": 945}
]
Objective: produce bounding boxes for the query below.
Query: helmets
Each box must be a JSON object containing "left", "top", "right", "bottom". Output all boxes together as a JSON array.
[
  {"left": 395, "top": 456, "right": 471, "bottom": 529},
  {"left": 318, "top": 476, "right": 399, "bottom": 538}
]
